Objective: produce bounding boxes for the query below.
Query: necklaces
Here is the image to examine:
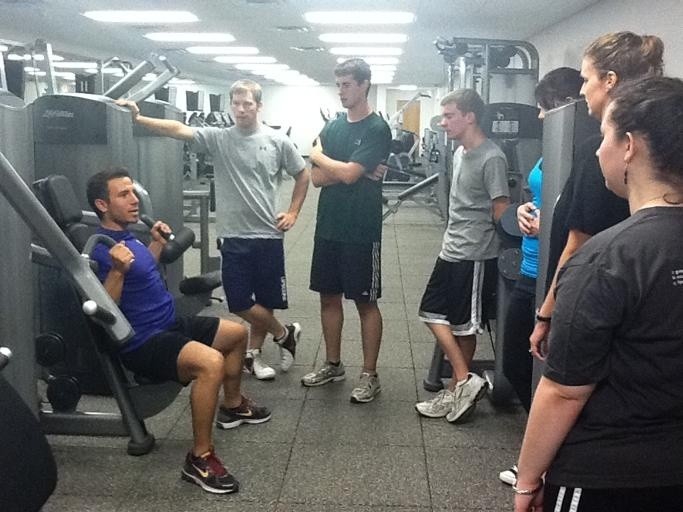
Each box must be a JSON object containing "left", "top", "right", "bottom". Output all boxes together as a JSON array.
[{"left": 642, "top": 192, "right": 678, "bottom": 204}]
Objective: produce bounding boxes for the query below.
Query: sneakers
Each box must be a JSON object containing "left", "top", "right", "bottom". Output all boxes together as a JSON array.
[
  {"left": 445, "top": 372, "right": 488, "bottom": 424},
  {"left": 414, "top": 389, "right": 453, "bottom": 417},
  {"left": 300, "top": 360, "right": 345, "bottom": 387},
  {"left": 350, "top": 372, "right": 380, "bottom": 403},
  {"left": 181, "top": 444, "right": 239, "bottom": 494},
  {"left": 499, "top": 463, "right": 519, "bottom": 486},
  {"left": 272, "top": 322, "right": 301, "bottom": 372},
  {"left": 216, "top": 395, "right": 272, "bottom": 429},
  {"left": 241, "top": 348, "right": 275, "bottom": 380}
]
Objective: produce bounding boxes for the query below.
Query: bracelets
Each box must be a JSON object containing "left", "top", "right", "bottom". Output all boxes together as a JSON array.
[
  {"left": 532, "top": 308, "right": 551, "bottom": 322},
  {"left": 510, "top": 478, "right": 543, "bottom": 494}
]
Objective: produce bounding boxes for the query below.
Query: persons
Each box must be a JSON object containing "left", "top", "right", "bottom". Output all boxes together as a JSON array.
[
  {"left": 523, "top": 29, "right": 666, "bottom": 363},
  {"left": 308, "top": 56, "right": 393, "bottom": 403},
  {"left": 81, "top": 166, "right": 272, "bottom": 495},
  {"left": 413, "top": 88, "right": 510, "bottom": 425},
  {"left": 509, "top": 76, "right": 682, "bottom": 510},
  {"left": 110, "top": 79, "right": 310, "bottom": 381},
  {"left": 497, "top": 67, "right": 586, "bottom": 489}
]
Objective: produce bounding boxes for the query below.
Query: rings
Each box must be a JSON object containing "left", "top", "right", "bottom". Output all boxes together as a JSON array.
[{"left": 528, "top": 349, "right": 532, "bottom": 352}]
